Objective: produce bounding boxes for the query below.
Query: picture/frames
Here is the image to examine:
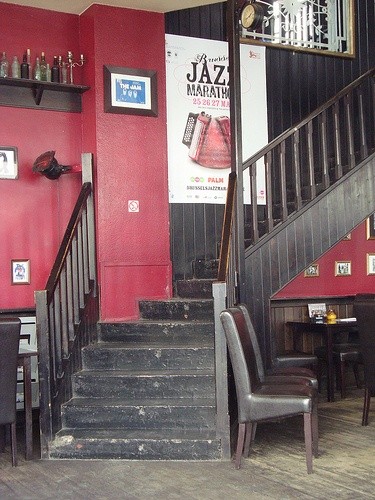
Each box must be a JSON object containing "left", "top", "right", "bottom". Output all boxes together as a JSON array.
[
  {"left": 366, "top": 252, "right": 375, "bottom": 276},
  {"left": 103, "top": 64, "right": 158, "bottom": 118},
  {"left": 0, "top": 146, "right": 19, "bottom": 180},
  {"left": 11, "top": 259, "right": 30, "bottom": 285},
  {"left": 366, "top": 212, "right": 375, "bottom": 241},
  {"left": 304, "top": 264, "right": 320, "bottom": 277},
  {"left": 334, "top": 261, "right": 351, "bottom": 277}
]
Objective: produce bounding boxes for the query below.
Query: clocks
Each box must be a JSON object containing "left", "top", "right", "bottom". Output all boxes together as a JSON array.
[{"left": 241, "top": 3, "right": 264, "bottom": 29}]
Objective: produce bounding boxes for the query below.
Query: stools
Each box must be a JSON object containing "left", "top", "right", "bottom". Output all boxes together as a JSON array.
[{"left": 314, "top": 345, "right": 362, "bottom": 399}]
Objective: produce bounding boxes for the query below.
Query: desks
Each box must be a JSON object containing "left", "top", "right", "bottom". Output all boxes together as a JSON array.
[
  {"left": 286, "top": 317, "right": 357, "bottom": 402},
  {"left": 19, "top": 347, "right": 38, "bottom": 459}
]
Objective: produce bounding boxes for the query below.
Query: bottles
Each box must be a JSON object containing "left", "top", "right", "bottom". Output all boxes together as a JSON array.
[
  {"left": 326, "top": 309, "right": 336, "bottom": 320},
  {"left": 58, "top": 55, "right": 63, "bottom": 83},
  {"left": 62, "top": 62, "right": 67, "bottom": 84},
  {"left": 47, "top": 64, "right": 52, "bottom": 82},
  {"left": 52, "top": 55, "right": 59, "bottom": 83},
  {"left": 0, "top": 52, "right": 9, "bottom": 77},
  {"left": 11, "top": 55, "right": 21, "bottom": 78},
  {"left": 21, "top": 54, "right": 29, "bottom": 79},
  {"left": 33, "top": 57, "right": 41, "bottom": 81},
  {"left": 41, "top": 52, "right": 47, "bottom": 81},
  {"left": 27, "top": 49, "right": 32, "bottom": 79}
]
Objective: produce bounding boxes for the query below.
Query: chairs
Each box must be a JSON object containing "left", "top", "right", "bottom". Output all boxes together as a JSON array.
[
  {"left": 219, "top": 307, "right": 318, "bottom": 475},
  {"left": 236, "top": 303, "right": 318, "bottom": 440},
  {"left": 355, "top": 293, "right": 375, "bottom": 426},
  {"left": 0, "top": 317, "right": 21, "bottom": 467}
]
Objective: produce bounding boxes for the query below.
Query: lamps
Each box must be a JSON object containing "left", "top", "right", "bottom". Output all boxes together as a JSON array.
[{"left": 32, "top": 150, "right": 72, "bottom": 180}]
